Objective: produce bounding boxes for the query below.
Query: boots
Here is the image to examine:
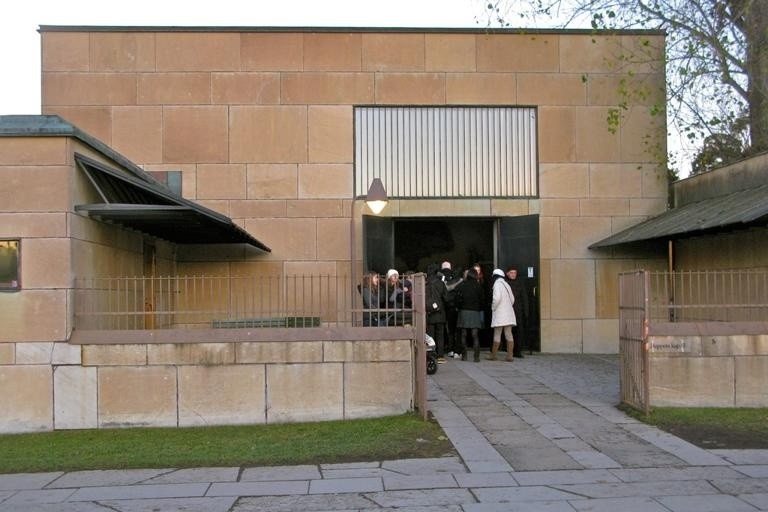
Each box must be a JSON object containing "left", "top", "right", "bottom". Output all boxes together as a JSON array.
[
  {"left": 506, "top": 341, "right": 514, "bottom": 361},
  {"left": 484, "top": 341, "right": 500, "bottom": 360}
]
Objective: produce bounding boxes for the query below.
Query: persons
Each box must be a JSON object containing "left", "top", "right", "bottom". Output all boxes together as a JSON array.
[{"left": 363, "top": 258, "right": 529, "bottom": 364}]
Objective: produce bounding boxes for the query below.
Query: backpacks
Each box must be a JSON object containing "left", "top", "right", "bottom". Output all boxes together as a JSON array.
[{"left": 426, "top": 278, "right": 443, "bottom": 315}]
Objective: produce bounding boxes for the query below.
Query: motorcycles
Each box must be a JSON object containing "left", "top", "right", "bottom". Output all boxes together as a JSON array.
[{"left": 425, "top": 302, "right": 441, "bottom": 376}]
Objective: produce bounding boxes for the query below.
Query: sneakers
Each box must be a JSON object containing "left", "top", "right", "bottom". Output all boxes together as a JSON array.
[{"left": 436, "top": 348, "right": 481, "bottom": 364}]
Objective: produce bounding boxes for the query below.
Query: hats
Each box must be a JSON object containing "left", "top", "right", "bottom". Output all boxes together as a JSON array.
[
  {"left": 441, "top": 261, "right": 451, "bottom": 271},
  {"left": 387, "top": 269, "right": 411, "bottom": 288},
  {"left": 493, "top": 268, "right": 506, "bottom": 278}
]
